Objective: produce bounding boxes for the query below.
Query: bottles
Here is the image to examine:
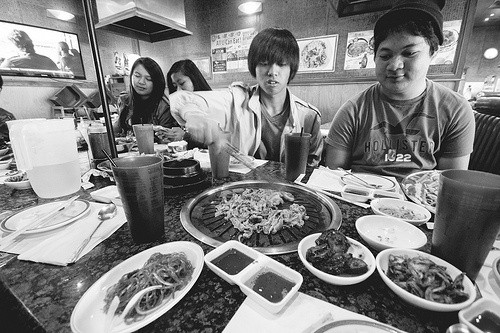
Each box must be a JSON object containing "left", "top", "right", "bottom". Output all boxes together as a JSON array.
[
  {"left": 87, "top": 122, "right": 112, "bottom": 164},
  {"left": 69, "top": 240, "right": 204, "bottom": 333}
]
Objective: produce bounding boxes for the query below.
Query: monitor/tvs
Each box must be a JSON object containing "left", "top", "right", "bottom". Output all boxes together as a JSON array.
[{"left": 0, "top": 19, "right": 87, "bottom": 80}]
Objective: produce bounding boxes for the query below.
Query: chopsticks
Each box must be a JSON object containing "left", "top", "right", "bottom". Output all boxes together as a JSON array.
[{"left": 1, "top": 195, "right": 81, "bottom": 250}]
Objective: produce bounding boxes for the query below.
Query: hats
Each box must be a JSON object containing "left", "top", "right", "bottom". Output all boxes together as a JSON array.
[{"left": 374, "top": 0, "right": 444, "bottom": 46}]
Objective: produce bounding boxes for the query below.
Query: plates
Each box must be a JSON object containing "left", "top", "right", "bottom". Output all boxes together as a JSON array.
[
  {"left": 355, "top": 215, "right": 428, "bottom": 252},
  {"left": 0, "top": 198, "right": 90, "bottom": 236},
  {"left": 402, "top": 170, "right": 442, "bottom": 214},
  {"left": 371, "top": 198, "right": 431, "bottom": 226},
  {"left": 340, "top": 173, "right": 396, "bottom": 191},
  {"left": 98, "top": 157, "right": 117, "bottom": 176},
  {"left": 298, "top": 233, "right": 376, "bottom": 286},
  {"left": 4, "top": 177, "right": 32, "bottom": 190},
  {"left": 204, "top": 240, "right": 304, "bottom": 316},
  {"left": 374, "top": 248, "right": 477, "bottom": 311}
]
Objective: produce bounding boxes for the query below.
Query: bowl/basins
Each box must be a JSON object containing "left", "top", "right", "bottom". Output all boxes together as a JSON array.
[{"left": 457, "top": 297, "right": 500, "bottom": 333}]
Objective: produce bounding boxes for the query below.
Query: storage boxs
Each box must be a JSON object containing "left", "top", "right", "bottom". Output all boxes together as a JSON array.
[{"left": 49, "top": 82, "right": 116, "bottom": 114}]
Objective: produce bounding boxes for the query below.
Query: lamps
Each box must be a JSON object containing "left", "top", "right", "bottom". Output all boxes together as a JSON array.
[
  {"left": 46, "top": 7, "right": 75, "bottom": 22},
  {"left": 236, "top": 1, "right": 264, "bottom": 15}
]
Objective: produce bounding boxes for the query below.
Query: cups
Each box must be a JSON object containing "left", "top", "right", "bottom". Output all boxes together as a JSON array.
[
  {"left": 132, "top": 123, "right": 155, "bottom": 155},
  {"left": 283, "top": 132, "right": 311, "bottom": 183},
  {"left": 21, "top": 120, "right": 82, "bottom": 199},
  {"left": 6, "top": 118, "right": 42, "bottom": 174},
  {"left": 208, "top": 131, "right": 231, "bottom": 187},
  {"left": 431, "top": 169, "right": 500, "bottom": 285},
  {"left": 110, "top": 157, "right": 165, "bottom": 243}
]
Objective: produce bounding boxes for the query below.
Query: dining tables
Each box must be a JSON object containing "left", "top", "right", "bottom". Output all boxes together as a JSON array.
[{"left": 0, "top": 150, "right": 456, "bottom": 333}]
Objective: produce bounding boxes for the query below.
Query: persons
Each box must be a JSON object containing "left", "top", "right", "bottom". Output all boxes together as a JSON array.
[
  {"left": 58, "top": 41, "right": 82, "bottom": 74},
  {"left": 0, "top": 76, "right": 15, "bottom": 142},
  {"left": 170, "top": 29, "right": 324, "bottom": 168},
  {"left": 114, "top": 58, "right": 176, "bottom": 138},
  {"left": 154, "top": 60, "right": 212, "bottom": 150},
  {"left": 0, "top": 29, "right": 59, "bottom": 76},
  {"left": 326, "top": 0, "right": 475, "bottom": 179}
]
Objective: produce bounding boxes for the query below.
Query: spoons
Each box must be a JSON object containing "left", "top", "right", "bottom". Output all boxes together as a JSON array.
[{"left": 66, "top": 202, "right": 117, "bottom": 264}]
